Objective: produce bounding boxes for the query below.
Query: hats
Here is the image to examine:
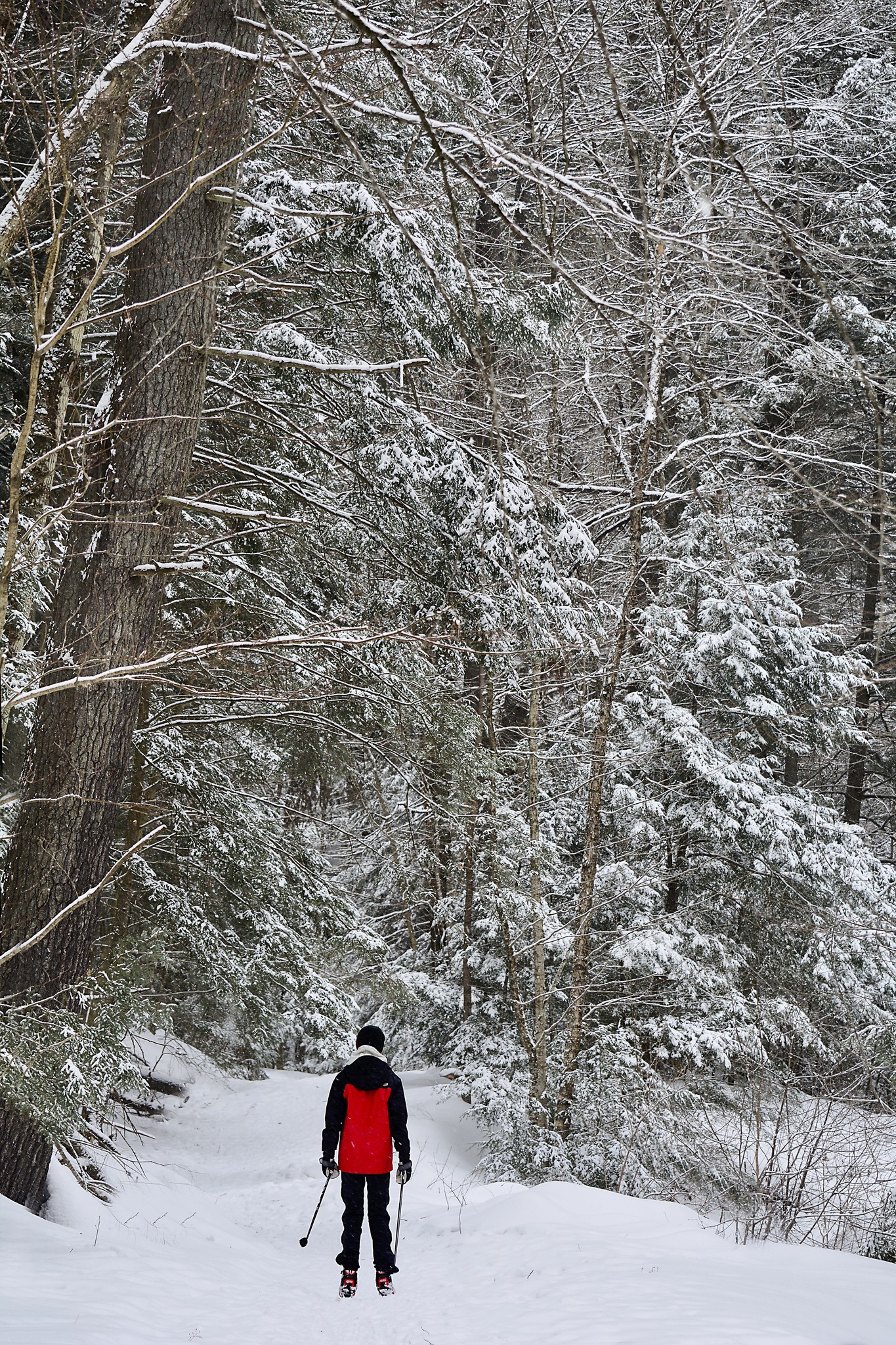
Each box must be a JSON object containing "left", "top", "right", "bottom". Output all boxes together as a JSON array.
[{"left": 355, "top": 1025, "right": 385, "bottom": 1053}]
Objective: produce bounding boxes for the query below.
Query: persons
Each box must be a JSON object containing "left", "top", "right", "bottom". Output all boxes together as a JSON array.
[{"left": 319, "top": 1026, "right": 412, "bottom": 1298}]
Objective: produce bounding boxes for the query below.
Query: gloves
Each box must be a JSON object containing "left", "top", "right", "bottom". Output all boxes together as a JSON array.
[
  {"left": 396, "top": 1160, "right": 412, "bottom": 1184},
  {"left": 319, "top": 1150, "right": 339, "bottom": 1179}
]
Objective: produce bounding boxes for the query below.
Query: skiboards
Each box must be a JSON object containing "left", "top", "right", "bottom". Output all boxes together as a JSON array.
[{"left": 323, "top": 1289, "right": 400, "bottom": 1306}]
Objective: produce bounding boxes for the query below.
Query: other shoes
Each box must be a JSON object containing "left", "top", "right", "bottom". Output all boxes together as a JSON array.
[
  {"left": 339, "top": 1270, "right": 357, "bottom": 1297},
  {"left": 375, "top": 1270, "right": 394, "bottom": 1296}
]
